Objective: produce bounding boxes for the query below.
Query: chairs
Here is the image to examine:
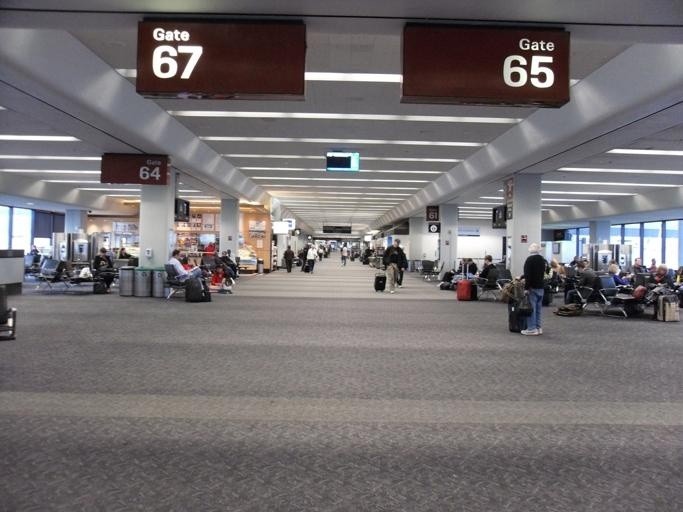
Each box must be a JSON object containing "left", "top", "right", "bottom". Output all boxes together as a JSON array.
[
  {"left": 21, "top": 240, "right": 241, "bottom": 306},
  {"left": 0, "top": 286, "right": 18, "bottom": 342},
  {"left": 415, "top": 252, "right": 683, "bottom": 318}
]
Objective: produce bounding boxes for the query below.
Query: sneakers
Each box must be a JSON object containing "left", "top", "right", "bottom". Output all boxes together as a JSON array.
[{"left": 523, "top": 329, "right": 542, "bottom": 336}]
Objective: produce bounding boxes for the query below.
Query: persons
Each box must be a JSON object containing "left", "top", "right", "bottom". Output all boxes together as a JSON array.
[
  {"left": 26, "top": 244, "right": 36, "bottom": 261},
  {"left": 284, "top": 245, "right": 294, "bottom": 273},
  {"left": 117, "top": 248, "right": 133, "bottom": 259},
  {"left": 544, "top": 256, "right": 596, "bottom": 305},
  {"left": 381, "top": 239, "right": 406, "bottom": 296},
  {"left": 609, "top": 258, "right": 682, "bottom": 290},
  {"left": 340, "top": 242, "right": 347, "bottom": 265},
  {"left": 298, "top": 241, "right": 330, "bottom": 271},
  {"left": 168, "top": 241, "right": 240, "bottom": 290},
  {"left": 517, "top": 242, "right": 549, "bottom": 337},
  {"left": 93, "top": 248, "right": 113, "bottom": 291},
  {"left": 437, "top": 255, "right": 497, "bottom": 291}
]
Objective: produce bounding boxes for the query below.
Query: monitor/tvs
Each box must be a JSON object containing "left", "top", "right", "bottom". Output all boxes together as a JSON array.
[{"left": 325, "top": 151, "right": 360, "bottom": 172}]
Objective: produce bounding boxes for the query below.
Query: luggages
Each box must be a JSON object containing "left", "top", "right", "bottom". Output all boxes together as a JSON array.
[
  {"left": 94, "top": 276, "right": 105, "bottom": 293},
  {"left": 458, "top": 263, "right": 471, "bottom": 300},
  {"left": 375, "top": 267, "right": 387, "bottom": 291},
  {"left": 658, "top": 292, "right": 680, "bottom": 322},
  {"left": 507, "top": 277, "right": 526, "bottom": 333},
  {"left": 543, "top": 276, "right": 552, "bottom": 306},
  {"left": 184, "top": 276, "right": 202, "bottom": 301}
]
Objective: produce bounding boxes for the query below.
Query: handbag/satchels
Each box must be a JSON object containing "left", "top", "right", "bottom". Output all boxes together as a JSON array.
[
  {"left": 201, "top": 282, "right": 211, "bottom": 302},
  {"left": 554, "top": 303, "right": 582, "bottom": 316},
  {"left": 516, "top": 295, "right": 532, "bottom": 316}
]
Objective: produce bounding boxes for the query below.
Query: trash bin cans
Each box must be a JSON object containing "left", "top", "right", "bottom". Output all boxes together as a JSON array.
[
  {"left": 120, "top": 268, "right": 134, "bottom": 296},
  {"left": 152, "top": 269, "right": 170, "bottom": 297},
  {"left": 257, "top": 259, "right": 264, "bottom": 273},
  {"left": 134, "top": 269, "right": 152, "bottom": 297}
]
